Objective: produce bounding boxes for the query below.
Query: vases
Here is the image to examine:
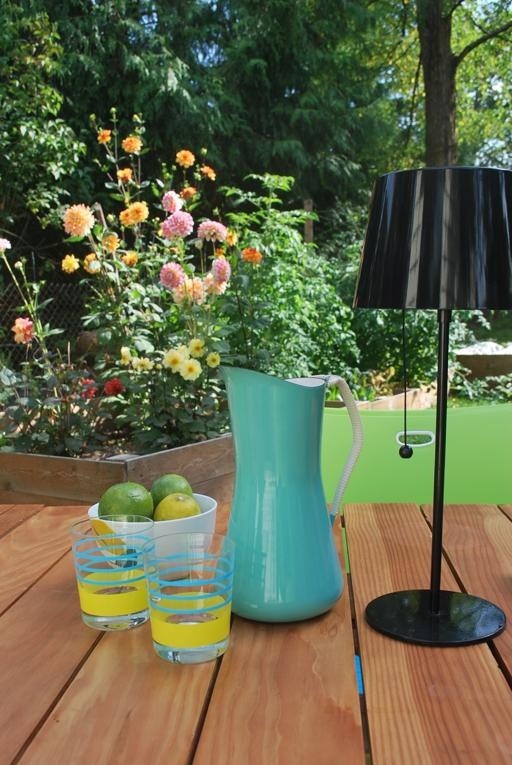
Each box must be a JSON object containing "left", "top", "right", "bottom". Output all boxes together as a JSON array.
[{"left": 0, "top": 432, "right": 235, "bottom": 506}]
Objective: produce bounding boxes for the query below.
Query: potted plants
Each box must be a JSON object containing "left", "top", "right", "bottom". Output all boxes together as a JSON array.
[{"left": 213, "top": 172, "right": 456, "bottom": 411}]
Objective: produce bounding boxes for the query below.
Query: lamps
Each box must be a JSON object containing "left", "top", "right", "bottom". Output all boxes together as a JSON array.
[{"left": 355, "top": 167, "right": 512, "bottom": 647}]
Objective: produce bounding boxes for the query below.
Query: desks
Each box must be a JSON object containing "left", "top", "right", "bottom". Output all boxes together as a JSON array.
[{"left": 0, "top": 504, "right": 512, "bottom": 765}]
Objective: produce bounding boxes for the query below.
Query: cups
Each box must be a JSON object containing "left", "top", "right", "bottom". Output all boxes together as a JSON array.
[{"left": 71, "top": 514, "right": 235, "bottom": 665}]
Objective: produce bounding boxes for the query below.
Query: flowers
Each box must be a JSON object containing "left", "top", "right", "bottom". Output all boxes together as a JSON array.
[{"left": 0, "top": 108, "right": 263, "bottom": 457}]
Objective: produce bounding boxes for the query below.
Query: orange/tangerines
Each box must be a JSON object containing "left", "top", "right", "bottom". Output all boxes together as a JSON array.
[
  {"left": 98, "top": 481, "right": 154, "bottom": 522},
  {"left": 151, "top": 474, "right": 193, "bottom": 506},
  {"left": 153, "top": 493, "right": 200, "bottom": 522}
]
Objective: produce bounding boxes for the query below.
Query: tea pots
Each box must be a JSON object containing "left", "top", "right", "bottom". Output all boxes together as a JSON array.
[{"left": 214, "top": 363, "right": 363, "bottom": 626}]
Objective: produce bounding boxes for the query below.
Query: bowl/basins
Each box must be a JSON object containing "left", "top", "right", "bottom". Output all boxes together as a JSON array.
[{"left": 75, "top": 492, "right": 221, "bottom": 581}]
